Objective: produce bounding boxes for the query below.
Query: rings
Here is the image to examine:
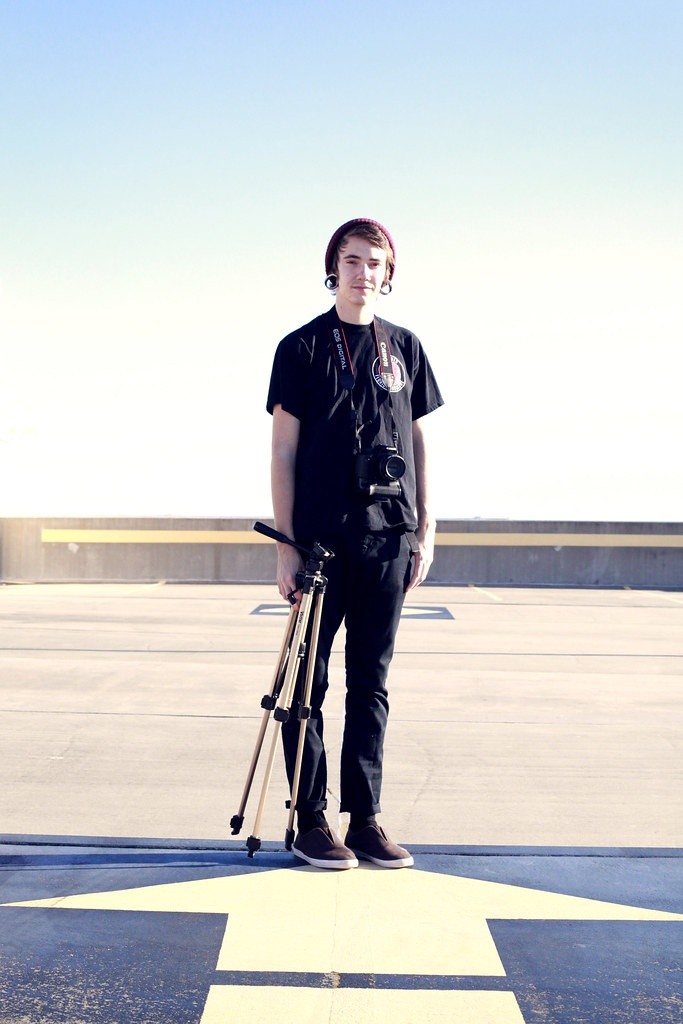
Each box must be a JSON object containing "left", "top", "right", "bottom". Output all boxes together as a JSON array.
[{"left": 292, "top": 595, "right": 294, "bottom": 597}]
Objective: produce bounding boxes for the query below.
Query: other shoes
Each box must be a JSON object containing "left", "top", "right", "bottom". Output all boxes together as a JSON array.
[
  {"left": 292, "top": 825, "right": 359, "bottom": 869},
  {"left": 344, "top": 820, "right": 414, "bottom": 868}
]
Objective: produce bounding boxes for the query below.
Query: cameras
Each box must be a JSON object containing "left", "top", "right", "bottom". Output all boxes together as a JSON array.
[{"left": 366, "top": 446, "right": 406, "bottom": 497}]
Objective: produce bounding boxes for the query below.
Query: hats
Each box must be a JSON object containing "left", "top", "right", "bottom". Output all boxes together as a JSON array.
[{"left": 321, "top": 219, "right": 397, "bottom": 288}]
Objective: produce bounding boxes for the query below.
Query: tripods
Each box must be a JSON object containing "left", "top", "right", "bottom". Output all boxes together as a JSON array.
[{"left": 230, "top": 521, "right": 335, "bottom": 858}]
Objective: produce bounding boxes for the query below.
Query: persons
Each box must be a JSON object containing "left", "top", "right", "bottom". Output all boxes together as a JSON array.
[{"left": 265, "top": 218, "right": 447, "bottom": 869}]
